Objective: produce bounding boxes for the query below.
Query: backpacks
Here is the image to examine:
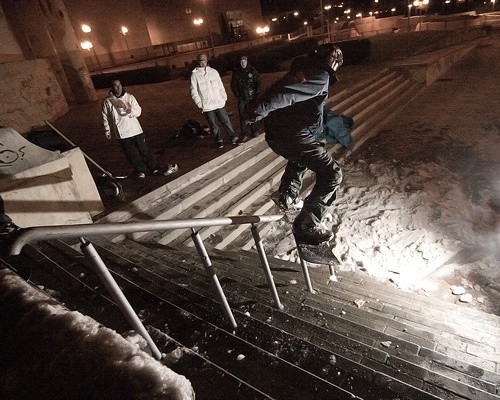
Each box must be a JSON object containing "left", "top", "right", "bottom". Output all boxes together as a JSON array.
[{"left": 180, "top": 119, "right": 206, "bottom": 140}]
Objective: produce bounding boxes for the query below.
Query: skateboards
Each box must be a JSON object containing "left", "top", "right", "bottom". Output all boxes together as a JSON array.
[{"left": 270, "top": 189, "right": 341, "bottom": 266}]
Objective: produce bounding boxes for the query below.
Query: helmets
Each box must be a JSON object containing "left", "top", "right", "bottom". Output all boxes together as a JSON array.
[{"left": 316, "top": 43, "right": 343, "bottom": 72}]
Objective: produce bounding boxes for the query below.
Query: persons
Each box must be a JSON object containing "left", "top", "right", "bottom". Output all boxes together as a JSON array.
[
  {"left": 242, "top": 43, "right": 343, "bottom": 244},
  {"left": 101, "top": 79, "right": 158, "bottom": 178},
  {"left": 190, "top": 53, "right": 243, "bottom": 149},
  {"left": 231, "top": 56, "right": 261, "bottom": 141}
]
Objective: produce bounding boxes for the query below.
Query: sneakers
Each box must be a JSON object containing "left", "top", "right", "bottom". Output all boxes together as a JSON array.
[
  {"left": 163, "top": 163, "right": 178, "bottom": 176},
  {"left": 198, "top": 54, "right": 208, "bottom": 62},
  {"left": 150, "top": 167, "right": 158, "bottom": 176},
  {"left": 293, "top": 210, "right": 334, "bottom": 243},
  {"left": 240, "top": 56, "right": 247, "bottom": 61},
  {"left": 280, "top": 193, "right": 304, "bottom": 210},
  {"left": 137, "top": 172, "right": 145, "bottom": 180},
  {"left": 217, "top": 139, "right": 224, "bottom": 149},
  {"left": 232, "top": 136, "right": 244, "bottom": 146}
]
